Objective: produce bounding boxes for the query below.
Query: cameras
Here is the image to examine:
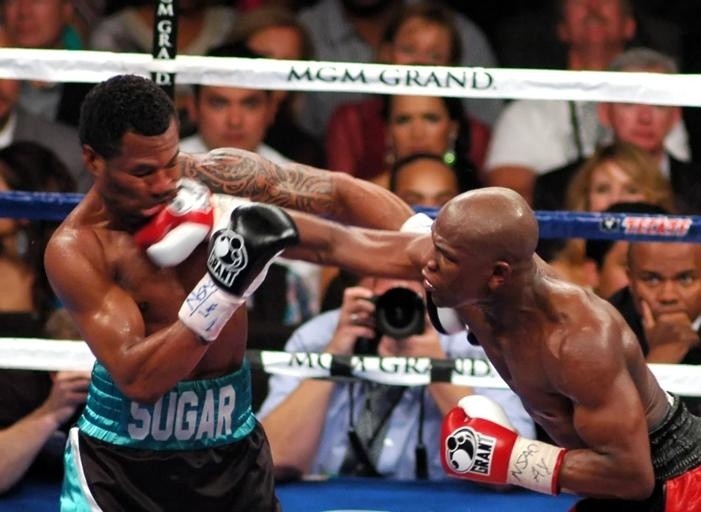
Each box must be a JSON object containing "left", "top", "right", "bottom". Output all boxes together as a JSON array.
[{"left": 354, "top": 284, "right": 427, "bottom": 357}]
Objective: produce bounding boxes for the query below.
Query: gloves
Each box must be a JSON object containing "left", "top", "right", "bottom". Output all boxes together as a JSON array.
[
  {"left": 133, "top": 177, "right": 252, "bottom": 272},
  {"left": 178, "top": 201, "right": 300, "bottom": 341},
  {"left": 438, "top": 394, "right": 567, "bottom": 497}
]
